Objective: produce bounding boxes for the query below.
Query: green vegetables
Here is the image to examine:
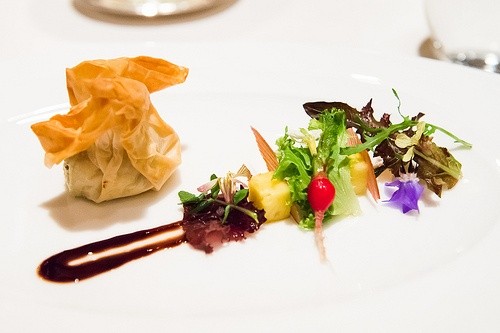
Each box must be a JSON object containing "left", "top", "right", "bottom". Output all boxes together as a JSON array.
[
  {"left": 337, "top": 87, "right": 472, "bottom": 156},
  {"left": 179, "top": 175, "right": 259, "bottom": 228}
]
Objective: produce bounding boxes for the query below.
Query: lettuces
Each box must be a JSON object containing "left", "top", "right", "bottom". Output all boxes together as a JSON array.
[{"left": 272, "top": 107, "right": 360, "bottom": 232}]
